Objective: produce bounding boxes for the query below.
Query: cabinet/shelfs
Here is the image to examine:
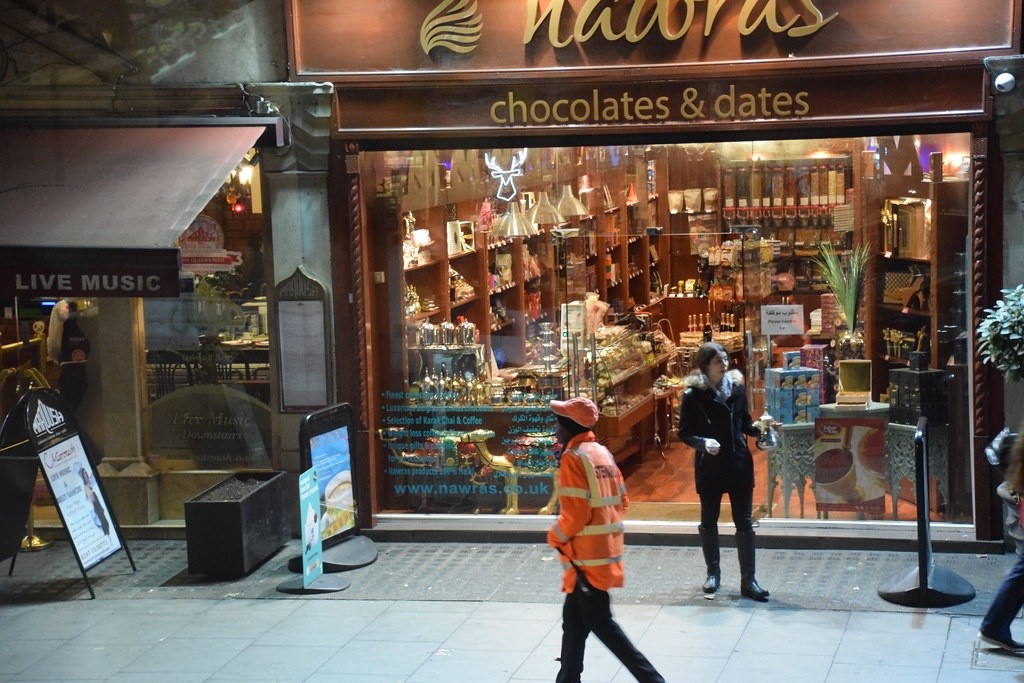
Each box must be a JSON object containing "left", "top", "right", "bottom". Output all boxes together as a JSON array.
[
  {"left": 859, "top": 150, "right": 970, "bottom": 514},
  {"left": 359, "top": 144, "right": 720, "bottom": 466},
  {"left": 597, "top": 352, "right": 673, "bottom": 466},
  {"left": 721, "top": 139, "right": 864, "bottom": 355}
]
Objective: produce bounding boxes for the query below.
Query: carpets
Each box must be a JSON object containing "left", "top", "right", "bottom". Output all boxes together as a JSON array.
[{"left": 624, "top": 501, "right": 777, "bottom": 525}]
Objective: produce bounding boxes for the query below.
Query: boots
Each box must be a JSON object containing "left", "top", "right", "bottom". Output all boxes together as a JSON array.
[
  {"left": 735, "top": 530, "right": 770, "bottom": 599},
  {"left": 697, "top": 525, "right": 721, "bottom": 594}
]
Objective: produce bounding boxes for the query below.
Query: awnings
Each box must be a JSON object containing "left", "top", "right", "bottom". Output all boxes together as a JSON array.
[{"left": 1, "top": 126, "right": 268, "bottom": 298}]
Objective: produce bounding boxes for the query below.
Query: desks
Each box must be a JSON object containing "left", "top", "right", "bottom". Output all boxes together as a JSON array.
[
  {"left": 223, "top": 339, "right": 269, "bottom": 379},
  {"left": 885, "top": 422, "right": 950, "bottom": 522},
  {"left": 766, "top": 423, "right": 828, "bottom": 519},
  {"left": 196, "top": 380, "right": 270, "bottom": 406}
]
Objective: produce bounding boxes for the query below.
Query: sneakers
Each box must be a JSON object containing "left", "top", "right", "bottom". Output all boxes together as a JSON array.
[{"left": 977, "top": 628, "right": 1023, "bottom": 652}]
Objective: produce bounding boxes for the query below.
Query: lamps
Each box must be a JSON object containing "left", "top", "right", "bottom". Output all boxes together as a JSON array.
[
  {"left": 486, "top": 149, "right": 540, "bottom": 237},
  {"left": 557, "top": 147, "right": 589, "bottom": 216},
  {"left": 524, "top": 148, "right": 566, "bottom": 224}
]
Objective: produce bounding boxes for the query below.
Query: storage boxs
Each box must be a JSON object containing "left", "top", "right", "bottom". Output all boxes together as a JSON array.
[
  {"left": 821, "top": 292, "right": 837, "bottom": 333},
  {"left": 764, "top": 351, "right": 820, "bottom": 424},
  {"left": 886, "top": 351, "right": 948, "bottom": 426},
  {"left": 800, "top": 344, "right": 830, "bottom": 405}
]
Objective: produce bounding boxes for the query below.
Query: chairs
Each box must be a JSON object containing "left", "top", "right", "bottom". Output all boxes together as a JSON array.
[
  {"left": 146, "top": 347, "right": 193, "bottom": 404},
  {"left": 198, "top": 344, "right": 251, "bottom": 380}
]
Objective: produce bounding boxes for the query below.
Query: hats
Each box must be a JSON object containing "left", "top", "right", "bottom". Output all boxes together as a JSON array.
[{"left": 548, "top": 397, "right": 599, "bottom": 429}]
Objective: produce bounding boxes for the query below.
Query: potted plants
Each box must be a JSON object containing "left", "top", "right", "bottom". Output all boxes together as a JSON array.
[{"left": 976, "top": 284, "right": 1024, "bottom": 556}]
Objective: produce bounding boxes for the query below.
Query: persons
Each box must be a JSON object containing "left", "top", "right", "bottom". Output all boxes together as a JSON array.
[
  {"left": 47, "top": 298, "right": 93, "bottom": 392},
  {"left": 678, "top": 342, "right": 782, "bottom": 598},
  {"left": 978, "top": 426, "right": 1024, "bottom": 652},
  {"left": 547, "top": 397, "right": 664, "bottom": 683},
  {"left": 79, "top": 467, "right": 110, "bottom": 535}
]
